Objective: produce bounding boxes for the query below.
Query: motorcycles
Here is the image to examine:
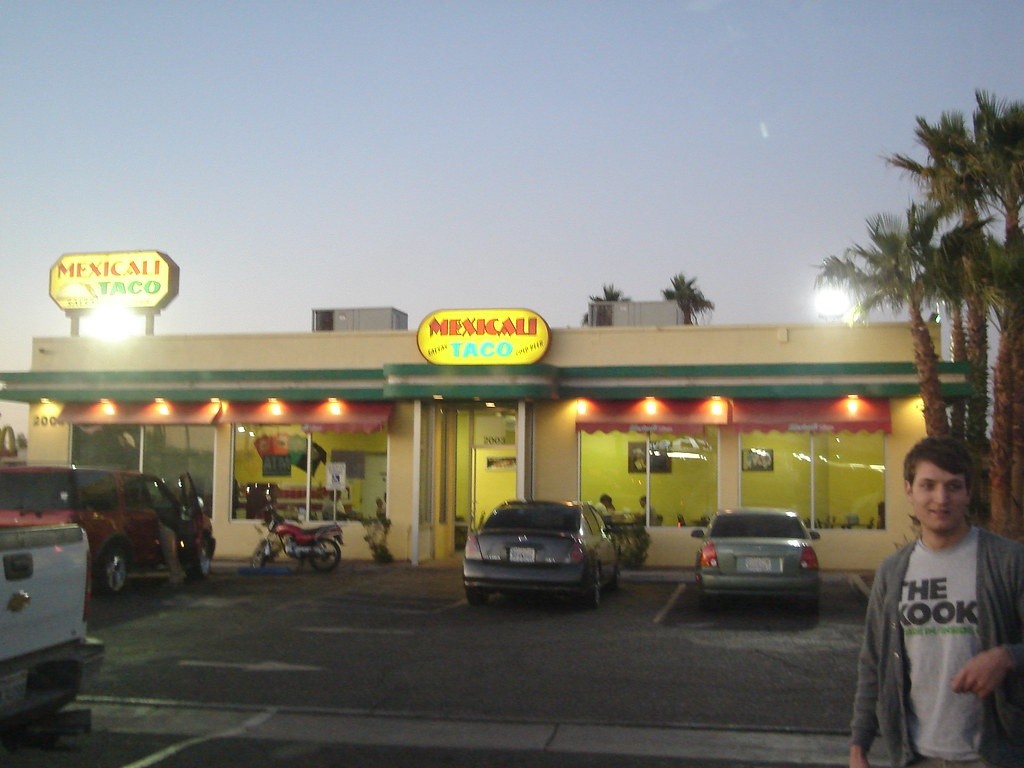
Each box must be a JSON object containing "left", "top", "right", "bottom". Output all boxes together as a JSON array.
[{"left": 249, "top": 507, "right": 343, "bottom": 575}]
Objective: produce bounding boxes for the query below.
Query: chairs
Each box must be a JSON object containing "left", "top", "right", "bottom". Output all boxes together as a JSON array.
[{"left": 656, "top": 514, "right": 874, "bottom": 529}]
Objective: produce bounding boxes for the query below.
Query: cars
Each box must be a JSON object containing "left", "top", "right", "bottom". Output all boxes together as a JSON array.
[
  {"left": 691, "top": 506, "right": 822, "bottom": 618},
  {"left": 462, "top": 500, "right": 624, "bottom": 611}
]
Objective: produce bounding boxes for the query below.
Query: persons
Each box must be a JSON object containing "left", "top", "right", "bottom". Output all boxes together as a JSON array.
[
  {"left": 634, "top": 495, "right": 661, "bottom": 525},
  {"left": 376, "top": 497, "right": 386, "bottom": 518},
  {"left": 159, "top": 521, "right": 187, "bottom": 581},
  {"left": 322, "top": 489, "right": 350, "bottom": 521},
  {"left": 594, "top": 494, "right": 612, "bottom": 520},
  {"left": 850, "top": 436, "right": 1024, "bottom": 768}
]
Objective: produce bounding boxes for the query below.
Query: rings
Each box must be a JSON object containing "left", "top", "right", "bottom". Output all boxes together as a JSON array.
[
  {"left": 985, "top": 684, "right": 992, "bottom": 692},
  {"left": 975, "top": 679, "right": 985, "bottom": 688}
]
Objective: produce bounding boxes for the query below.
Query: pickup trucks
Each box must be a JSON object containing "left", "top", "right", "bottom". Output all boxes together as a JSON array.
[{"left": 0, "top": 523, "right": 104, "bottom": 736}]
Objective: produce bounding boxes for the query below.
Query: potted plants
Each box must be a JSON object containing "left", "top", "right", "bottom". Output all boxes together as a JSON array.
[
  {"left": 358, "top": 514, "right": 394, "bottom": 564},
  {"left": 612, "top": 517, "right": 653, "bottom": 570}
]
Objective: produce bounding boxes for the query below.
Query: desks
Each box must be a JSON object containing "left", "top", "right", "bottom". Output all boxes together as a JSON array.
[{"left": 612, "top": 514, "right": 637, "bottom": 525}]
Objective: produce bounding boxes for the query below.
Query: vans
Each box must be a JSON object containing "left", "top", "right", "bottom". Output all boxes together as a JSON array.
[{"left": 0, "top": 463, "right": 216, "bottom": 597}]
